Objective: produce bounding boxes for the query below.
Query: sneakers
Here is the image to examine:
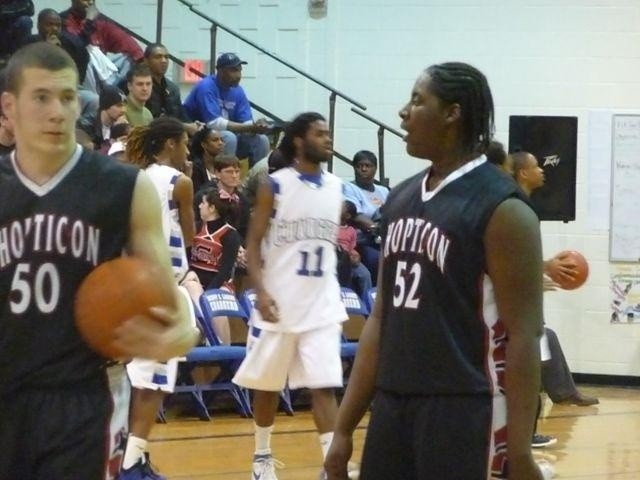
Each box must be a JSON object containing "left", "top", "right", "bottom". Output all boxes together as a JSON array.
[
  {"left": 250, "top": 454, "right": 286, "bottom": 480},
  {"left": 321, "top": 461, "right": 361, "bottom": 480},
  {"left": 531, "top": 433, "right": 558, "bottom": 447},
  {"left": 117, "top": 452, "right": 166, "bottom": 479}
]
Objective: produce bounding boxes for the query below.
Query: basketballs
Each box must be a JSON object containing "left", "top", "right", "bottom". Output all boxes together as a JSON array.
[
  {"left": 76, "top": 256, "right": 179, "bottom": 362},
  {"left": 553, "top": 249, "right": 588, "bottom": 290}
]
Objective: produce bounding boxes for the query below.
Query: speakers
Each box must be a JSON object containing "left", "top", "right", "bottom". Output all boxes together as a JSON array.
[{"left": 508, "top": 115, "right": 579, "bottom": 223}]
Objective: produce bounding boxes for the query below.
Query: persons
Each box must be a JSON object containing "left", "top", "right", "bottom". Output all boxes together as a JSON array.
[
  {"left": 342, "top": 150, "right": 392, "bottom": 220},
  {"left": 184, "top": 188, "right": 242, "bottom": 347},
  {"left": 1, "top": 39, "right": 203, "bottom": 480},
  {"left": 331, "top": 200, "right": 374, "bottom": 318},
  {"left": 505, "top": 150, "right": 601, "bottom": 408},
  {"left": 325, "top": 60, "right": 543, "bottom": 480},
  {"left": 240, "top": 108, "right": 363, "bottom": 480},
  {"left": 190, "top": 152, "right": 250, "bottom": 250},
  {"left": 0, "top": 0, "right": 288, "bottom": 190},
  {"left": 111, "top": 116, "right": 202, "bottom": 480},
  {"left": 474, "top": 135, "right": 560, "bottom": 479}
]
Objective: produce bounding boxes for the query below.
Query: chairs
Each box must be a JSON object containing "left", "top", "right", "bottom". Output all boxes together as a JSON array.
[{"left": 142, "top": 284, "right": 386, "bottom": 425}]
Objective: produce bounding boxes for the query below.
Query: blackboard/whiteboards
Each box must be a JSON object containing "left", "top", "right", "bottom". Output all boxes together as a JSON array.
[{"left": 608, "top": 114, "right": 640, "bottom": 264}]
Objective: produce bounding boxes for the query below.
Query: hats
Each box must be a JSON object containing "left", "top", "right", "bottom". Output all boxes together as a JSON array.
[
  {"left": 217, "top": 53, "right": 248, "bottom": 68},
  {"left": 99, "top": 86, "right": 128, "bottom": 110}
]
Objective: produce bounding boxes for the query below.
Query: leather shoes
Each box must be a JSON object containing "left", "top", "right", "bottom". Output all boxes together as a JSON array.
[{"left": 557, "top": 392, "right": 599, "bottom": 407}]
